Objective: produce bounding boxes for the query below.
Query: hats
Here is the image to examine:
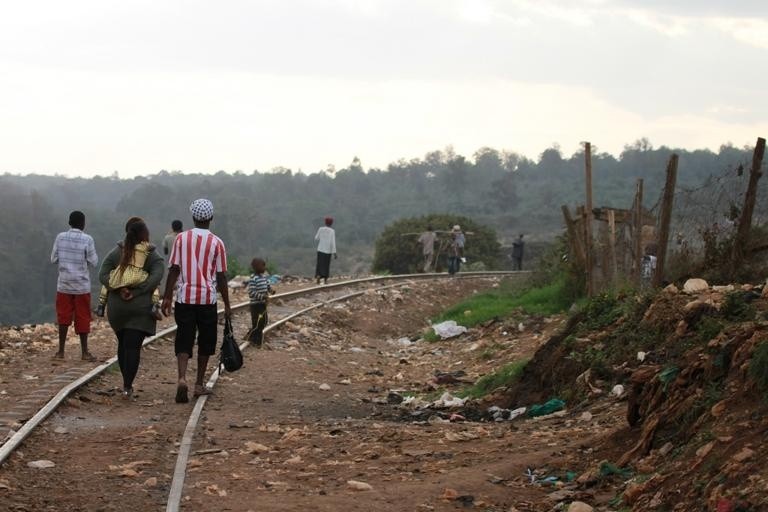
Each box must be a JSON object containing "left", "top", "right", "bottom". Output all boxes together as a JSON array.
[
  {"left": 325, "top": 216, "right": 333, "bottom": 224},
  {"left": 189, "top": 199, "right": 214, "bottom": 222}
]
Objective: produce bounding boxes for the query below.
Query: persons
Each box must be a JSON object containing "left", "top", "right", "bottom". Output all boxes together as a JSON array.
[
  {"left": 93, "top": 221, "right": 163, "bottom": 321},
  {"left": 454, "top": 225, "right": 467, "bottom": 271},
  {"left": 50, "top": 210, "right": 98, "bottom": 359},
  {"left": 445, "top": 233, "right": 460, "bottom": 276},
  {"left": 163, "top": 220, "right": 184, "bottom": 260},
  {"left": 99, "top": 217, "right": 166, "bottom": 402},
  {"left": 161, "top": 198, "right": 245, "bottom": 403},
  {"left": 241, "top": 256, "right": 275, "bottom": 346},
  {"left": 418, "top": 222, "right": 440, "bottom": 273},
  {"left": 314, "top": 216, "right": 337, "bottom": 284},
  {"left": 511, "top": 234, "right": 525, "bottom": 271},
  {"left": 639, "top": 242, "right": 659, "bottom": 284}
]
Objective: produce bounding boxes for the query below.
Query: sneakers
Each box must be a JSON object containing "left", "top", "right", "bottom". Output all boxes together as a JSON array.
[
  {"left": 149, "top": 303, "right": 162, "bottom": 320},
  {"left": 121, "top": 387, "right": 139, "bottom": 402},
  {"left": 193, "top": 382, "right": 212, "bottom": 397},
  {"left": 175, "top": 378, "right": 189, "bottom": 403},
  {"left": 92, "top": 305, "right": 105, "bottom": 317}
]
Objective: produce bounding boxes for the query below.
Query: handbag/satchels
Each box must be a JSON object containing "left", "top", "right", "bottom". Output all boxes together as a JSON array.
[{"left": 216, "top": 312, "right": 243, "bottom": 375}]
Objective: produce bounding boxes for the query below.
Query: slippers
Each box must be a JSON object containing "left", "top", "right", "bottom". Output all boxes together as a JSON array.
[
  {"left": 50, "top": 352, "right": 64, "bottom": 359},
  {"left": 80, "top": 354, "right": 98, "bottom": 362}
]
